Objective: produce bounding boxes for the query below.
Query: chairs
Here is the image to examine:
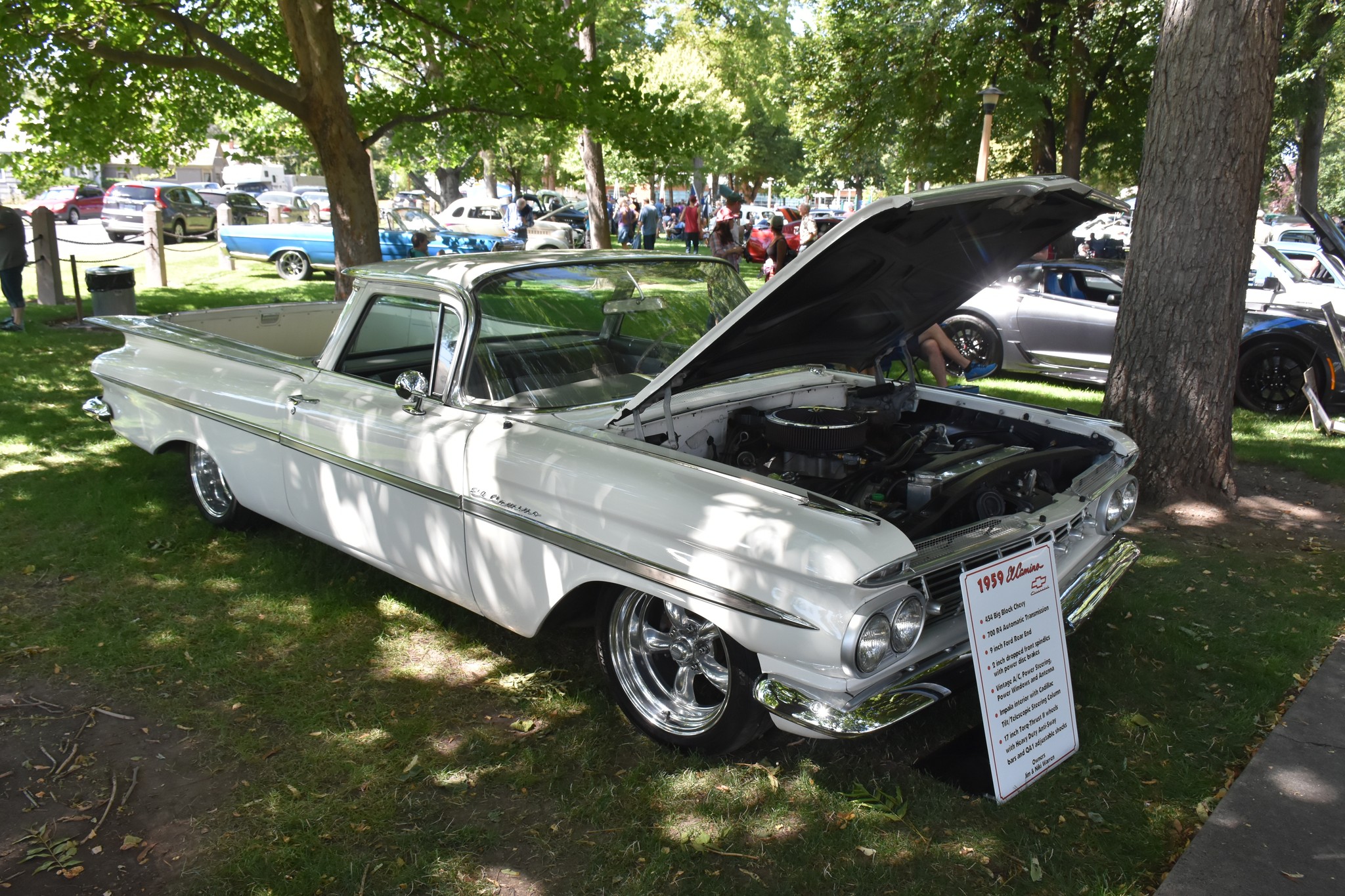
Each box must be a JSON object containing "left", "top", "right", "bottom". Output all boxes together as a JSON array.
[
  {"left": 1046, "top": 272, "right": 1066, "bottom": 297},
  {"left": 1061, "top": 272, "right": 1087, "bottom": 300},
  {"left": 881, "top": 348, "right": 923, "bottom": 384}
]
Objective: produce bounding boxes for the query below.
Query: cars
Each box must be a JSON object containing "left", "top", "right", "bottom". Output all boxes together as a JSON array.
[
  {"left": 80, "top": 174, "right": 1138, "bottom": 781},
  {"left": 20, "top": 185, "right": 106, "bottom": 225},
  {"left": 179, "top": 177, "right": 584, "bottom": 251},
  {"left": 939, "top": 193, "right": 1345, "bottom": 414},
  {"left": 709, "top": 203, "right": 848, "bottom": 263}
]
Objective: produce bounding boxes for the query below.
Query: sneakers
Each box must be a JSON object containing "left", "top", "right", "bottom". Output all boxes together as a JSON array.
[{"left": 964, "top": 361, "right": 998, "bottom": 382}]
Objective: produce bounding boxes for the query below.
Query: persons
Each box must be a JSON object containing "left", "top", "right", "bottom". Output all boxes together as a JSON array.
[
  {"left": 744, "top": 217, "right": 755, "bottom": 241},
  {"left": 617, "top": 197, "right": 639, "bottom": 249},
  {"left": 725, "top": 191, "right": 756, "bottom": 273},
  {"left": 407, "top": 230, "right": 446, "bottom": 258},
  {"left": 0, "top": 202, "right": 29, "bottom": 334},
  {"left": 584, "top": 200, "right": 591, "bottom": 249},
  {"left": 637, "top": 198, "right": 659, "bottom": 250},
  {"left": 760, "top": 216, "right": 788, "bottom": 285},
  {"left": 843, "top": 205, "right": 856, "bottom": 218},
  {"left": 606, "top": 194, "right": 620, "bottom": 235},
  {"left": 882, "top": 322, "right": 997, "bottom": 396},
  {"left": 797, "top": 203, "right": 818, "bottom": 256},
  {"left": 1089, "top": 232, "right": 1097, "bottom": 241},
  {"left": 654, "top": 198, "right": 722, "bottom": 248},
  {"left": 626, "top": 192, "right": 641, "bottom": 246},
  {"left": 708, "top": 207, "right": 744, "bottom": 331},
  {"left": 503, "top": 197, "right": 535, "bottom": 250},
  {"left": 679, "top": 196, "right": 703, "bottom": 254},
  {"left": 1075, "top": 243, "right": 1090, "bottom": 258}
]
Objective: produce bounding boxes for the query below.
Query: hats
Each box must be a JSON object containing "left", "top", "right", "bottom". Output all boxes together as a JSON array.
[
  {"left": 716, "top": 207, "right": 739, "bottom": 222},
  {"left": 767, "top": 216, "right": 784, "bottom": 229},
  {"left": 628, "top": 193, "right": 637, "bottom": 198}
]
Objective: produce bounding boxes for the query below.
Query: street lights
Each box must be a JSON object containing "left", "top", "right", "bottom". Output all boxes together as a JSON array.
[{"left": 766, "top": 177, "right": 775, "bottom": 209}]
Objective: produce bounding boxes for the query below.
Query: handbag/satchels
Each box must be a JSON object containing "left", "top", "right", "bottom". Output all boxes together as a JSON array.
[{"left": 628, "top": 225, "right": 634, "bottom": 238}]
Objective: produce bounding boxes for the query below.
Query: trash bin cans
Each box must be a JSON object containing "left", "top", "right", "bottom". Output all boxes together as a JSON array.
[{"left": 84, "top": 264, "right": 138, "bottom": 319}]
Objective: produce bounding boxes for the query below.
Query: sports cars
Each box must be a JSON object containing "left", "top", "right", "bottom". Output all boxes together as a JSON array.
[{"left": 217, "top": 200, "right": 526, "bottom": 280}]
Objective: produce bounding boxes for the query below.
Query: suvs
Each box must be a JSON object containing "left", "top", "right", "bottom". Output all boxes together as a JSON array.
[{"left": 100, "top": 180, "right": 216, "bottom": 244}]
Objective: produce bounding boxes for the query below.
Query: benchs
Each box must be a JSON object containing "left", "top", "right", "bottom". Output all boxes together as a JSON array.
[{"left": 371, "top": 345, "right": 619, "bottom": 401}]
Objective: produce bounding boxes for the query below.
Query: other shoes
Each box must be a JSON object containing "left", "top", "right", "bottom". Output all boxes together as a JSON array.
[{"left": 0, "top": 316, "right": 23, "bottom": 331}]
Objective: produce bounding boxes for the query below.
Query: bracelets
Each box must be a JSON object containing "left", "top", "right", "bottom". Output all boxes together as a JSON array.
[
  {"left": 743, "top": 225, "right": 746, "bottom": 229},
  {"left": 585, "top": 218, "right": 587, "bottom": 221}
]
